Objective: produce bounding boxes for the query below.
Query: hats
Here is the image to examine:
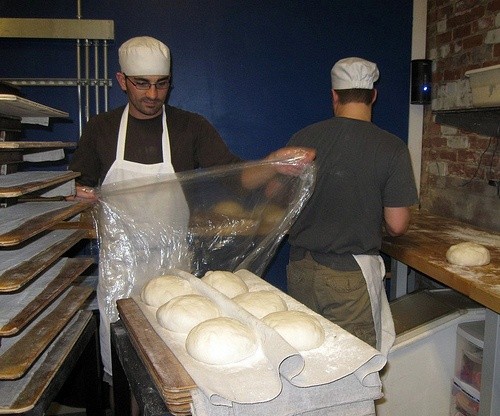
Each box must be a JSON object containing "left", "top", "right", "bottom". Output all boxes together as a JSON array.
[
  {"left": 118, "top": 37, "right": 172, "bottom": 77},
  {"left": 330, "top": 57, "right": 380, "bottom": 93}
]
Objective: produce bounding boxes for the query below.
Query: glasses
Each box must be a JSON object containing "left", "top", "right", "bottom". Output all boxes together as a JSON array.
[{"left": 126, "top": 78, "right": 172, "bottom": 90}]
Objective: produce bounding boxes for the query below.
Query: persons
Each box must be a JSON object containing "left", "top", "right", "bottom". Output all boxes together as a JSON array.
[
  {"left": 64, "top": 36, "right": 316, "bottom": 416},
  {"left": 263, "top": 57, "right": 418, "bottom": 350}
]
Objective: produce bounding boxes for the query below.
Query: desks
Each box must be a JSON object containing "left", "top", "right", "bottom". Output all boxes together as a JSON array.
[{"left": 378, "top": 209, "right": 500, "bottom": 416}]
